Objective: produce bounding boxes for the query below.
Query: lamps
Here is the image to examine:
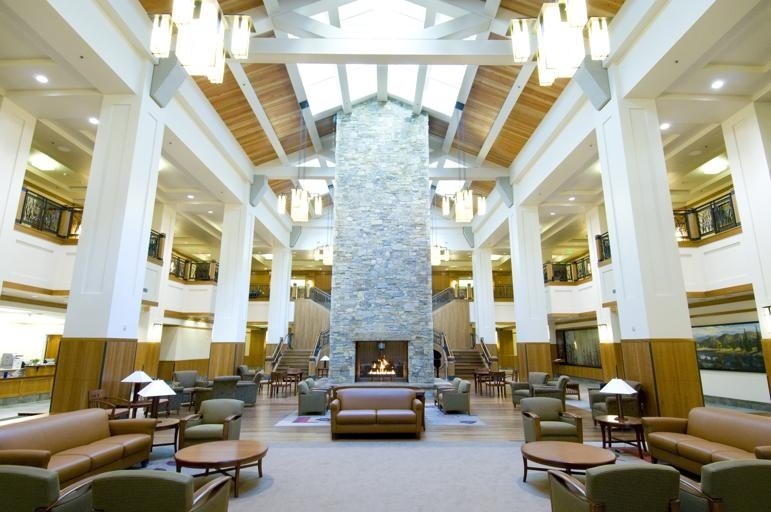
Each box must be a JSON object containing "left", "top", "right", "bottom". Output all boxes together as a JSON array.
[
  {"left": 441, "top": 108, "right": 488, "bottom": 224},
  {"left": 145, "top": 0, "right": 253, "bottom": 85},
  {"left": 275, "top": 103, "right": 323, "bottom": 224},
  {"left": 509, "top": 0, "right": 612, "bottom": 88}
]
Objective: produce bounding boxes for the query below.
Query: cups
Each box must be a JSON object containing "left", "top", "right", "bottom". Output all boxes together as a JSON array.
[{"left": 4, "top": 372, "right": 8, "bottom": 378}]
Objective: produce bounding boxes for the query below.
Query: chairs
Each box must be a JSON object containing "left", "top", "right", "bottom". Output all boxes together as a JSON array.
[
  {"left": 679, "top": 456, "right": 770, "bottom": 510},
  {"left": 546, "top": 461, "right": 684, "bottom": 512},
  {"left": 234, "top": 362, "right": 471, "bottom": 437},
  {"left": 0, "top": 364, "right": 234, "bottom": 512},
  {"left": 641, "top": 405, "right": 769, "bottom": 477},
  {"left": 520, "top": 395, "right": 583, "bottom": 441},
  {"left": 473, "top": 368, "right": 649, "bottom": 424}
]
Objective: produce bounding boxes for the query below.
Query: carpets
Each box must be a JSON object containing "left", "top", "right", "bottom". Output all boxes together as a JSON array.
[{"left": 126, "top": 437, "right": 652, "bottom": 512}]
